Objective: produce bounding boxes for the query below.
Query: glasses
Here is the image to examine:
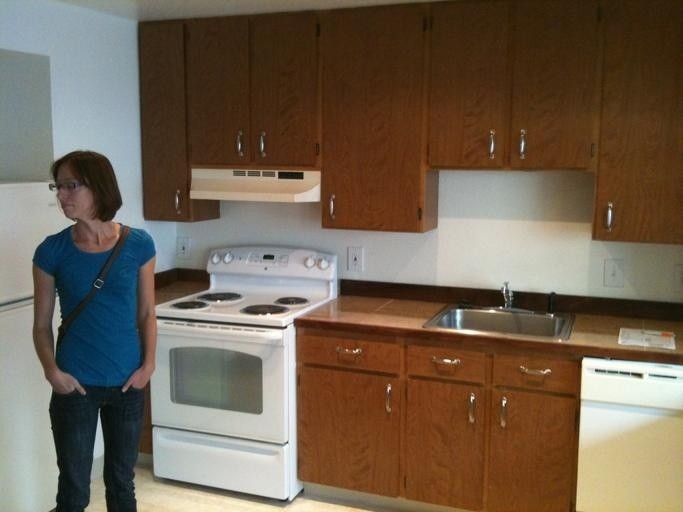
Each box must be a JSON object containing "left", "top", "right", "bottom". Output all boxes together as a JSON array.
[{"left": 49, "top": 181, "right": 84, "bottom": 193}]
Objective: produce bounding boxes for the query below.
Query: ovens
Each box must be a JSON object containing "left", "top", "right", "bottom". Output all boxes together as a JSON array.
[{"left": 149, "top": 319, "right": 301, "bottom": 503}]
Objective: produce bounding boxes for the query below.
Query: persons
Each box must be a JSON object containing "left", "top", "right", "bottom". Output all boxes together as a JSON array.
[{"left": 33, "top": 151, "right": 157, "bottom": 512}]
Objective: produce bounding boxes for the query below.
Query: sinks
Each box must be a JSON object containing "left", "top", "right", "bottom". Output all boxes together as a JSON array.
[{"left": 422, "top": 300, "right": 576, "bottom": 341}]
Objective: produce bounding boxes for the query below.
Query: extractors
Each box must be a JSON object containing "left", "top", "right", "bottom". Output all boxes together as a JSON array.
[{"left": 190, "top": 166, "right": 320, "bottom": 203}]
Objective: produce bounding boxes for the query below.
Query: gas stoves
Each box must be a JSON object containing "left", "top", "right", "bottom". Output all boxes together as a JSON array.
[{"left": 156, "top": 244, "right": 336, "bottom": 327}]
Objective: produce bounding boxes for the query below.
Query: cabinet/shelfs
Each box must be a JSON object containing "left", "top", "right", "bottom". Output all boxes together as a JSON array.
[
  {"left": 293, "top": 323, "right": 404, "bottom": 512},
  {"left": 404, "top": 334, "right": 581, "bottom": 511},
  {"left": 594, "top": 0, "right": 682, "bottom": 245},
  {"left": 428, "top": 0, "right": 594, "bottom": 171},
  {"left": 138, "top": 18, "right": 220, "bottom": 222},
  {"left": 319, "top": 10, "right": 437, "bottom": 234},
  {"left": 184, "top": 11, "right": 321, "bottom": 170}
]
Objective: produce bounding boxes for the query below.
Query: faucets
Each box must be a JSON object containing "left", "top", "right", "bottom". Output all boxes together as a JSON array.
[{"left": 501, "top": 283, "right": 514, "bottom": 307}]
